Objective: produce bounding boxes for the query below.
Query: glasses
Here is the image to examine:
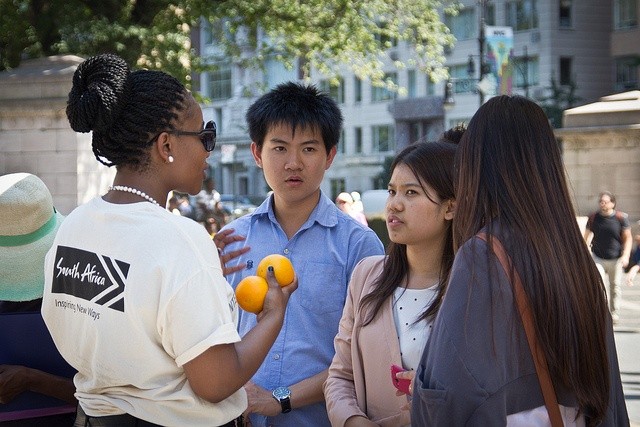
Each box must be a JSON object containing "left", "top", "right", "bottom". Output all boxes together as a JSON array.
[{"left": 148, "top": 119, "right": 219, "bottom": 152}]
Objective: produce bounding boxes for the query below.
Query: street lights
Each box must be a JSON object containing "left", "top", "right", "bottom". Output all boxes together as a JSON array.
[
  {"left": 468, "top": 45, "right": 529, "bottom": 99},
  {"left": 465, "top": 17, "right": 493, "bottom": 109}
]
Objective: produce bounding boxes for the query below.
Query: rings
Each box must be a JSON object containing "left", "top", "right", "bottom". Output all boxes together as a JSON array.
[{"left": 217, "top": 247, "right": 223, "bottom": 255}]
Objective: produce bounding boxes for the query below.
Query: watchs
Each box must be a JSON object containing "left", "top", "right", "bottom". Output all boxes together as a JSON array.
[{"left": 271, "top": 386, "right": 292, "bottom": 414}]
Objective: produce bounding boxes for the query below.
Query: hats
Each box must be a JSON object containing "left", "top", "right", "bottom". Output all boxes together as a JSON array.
[{"left": 1, "top": 172, "right": 67, "bottom": 305}]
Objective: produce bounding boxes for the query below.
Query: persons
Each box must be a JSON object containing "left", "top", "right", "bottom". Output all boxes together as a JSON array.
[
  {"left": 584, "top": 191, "right": 631, "bottom": 325},
  {"left": 40, "top": 52, "right": 299, "bottom": 426},
  {"left": 214, "top": 80, "right": 385, "bottom": 426},
  {"left": 336, "top": 191, "right": 352, "bottom": 228},
  {"left": 408, "top": 94, "right": 632, "bottom": 426},
  {"left": 0, "top": 172, "right": 79, "bottom": 426},
  {"left": 321, "top": 139, "right": 460, "bottom": 426},
  {"left": 352, "top": 191, "right": 369, "bottom": 230},
  {"left": 196, "top": 177, "right": 226, "bottom": 235}
]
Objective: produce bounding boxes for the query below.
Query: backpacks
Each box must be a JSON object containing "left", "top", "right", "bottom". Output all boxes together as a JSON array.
[
  {"left": 588, "top": 214, "right": 593, "bottom": 225},
  {"left": 616, "top": 211, "right": 624, "bottom": 222}
]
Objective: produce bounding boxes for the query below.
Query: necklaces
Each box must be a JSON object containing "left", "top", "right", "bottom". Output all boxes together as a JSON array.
[{"left": 107, "top": 185, "right": 164, "bottom": 207}]
